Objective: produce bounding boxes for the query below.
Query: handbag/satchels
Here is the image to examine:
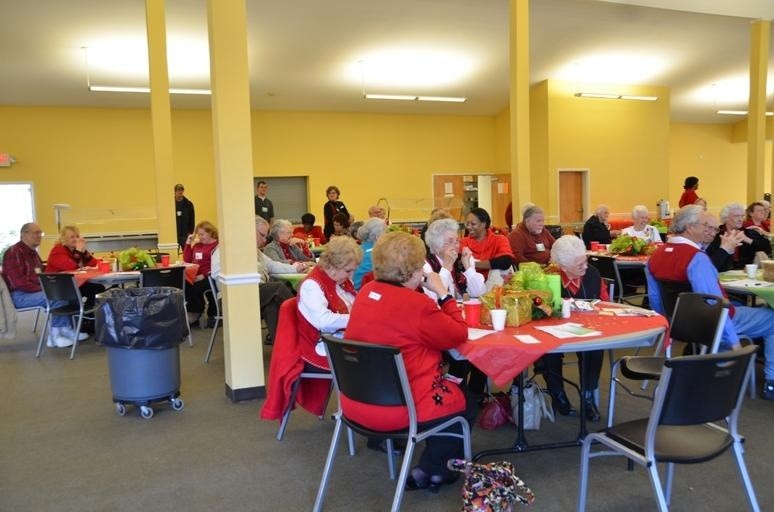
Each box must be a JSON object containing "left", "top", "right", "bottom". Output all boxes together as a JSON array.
[
  {"left": 476, "top": 390, "right": 515, "bottom": 431},
  {"left": 445, "top": 457, "right": 535, "bottom": 511},
  {"left": 509, "top": 377, "right": 555, "bottom": 431}
]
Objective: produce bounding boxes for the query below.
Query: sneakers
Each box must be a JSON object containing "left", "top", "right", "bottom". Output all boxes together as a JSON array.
[
  {"left": 45, "top": 325, "right": 89, "bottom": 348},
  {"left": 404, "top": 467, "right": 460, "bottom": 494},
  {"left": 760, "top": 380, "right": 774, "bottom": 400}
]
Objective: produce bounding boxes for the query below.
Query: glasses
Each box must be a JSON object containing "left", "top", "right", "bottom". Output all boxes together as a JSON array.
[{"left": 691, "top": 207, "right": 771, "bottom": 233}]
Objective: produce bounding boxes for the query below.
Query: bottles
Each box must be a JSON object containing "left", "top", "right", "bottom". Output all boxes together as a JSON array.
[
  {"left": 663, "top": 212, "right": 672, "bottom": 226},
  {"left": 305, "top": 233, "right": 314, "bottom": 249}
]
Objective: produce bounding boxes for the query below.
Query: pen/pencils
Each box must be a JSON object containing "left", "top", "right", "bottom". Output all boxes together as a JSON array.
[
  {"left": 745, "top": 283, "right": 764, "bottom": 285},
  {"left": 423, "top": 275, "right": 428, "bottom": 285}
]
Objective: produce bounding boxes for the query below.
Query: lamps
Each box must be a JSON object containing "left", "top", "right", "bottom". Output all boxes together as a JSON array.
[
  {"left": 361, "top": 88, "right": 468, "bottom": 104},
  {"left": 576, "top": 91, "right": 658, "bottom": 102},
  {"left": 717, "top": 109, "right": 773, "bottom": 117},
  {"left": 81, "top": 76, "right": 211, "bottom": 96}
]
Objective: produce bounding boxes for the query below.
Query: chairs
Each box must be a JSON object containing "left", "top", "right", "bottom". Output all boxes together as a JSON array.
[
  {"left": 313, "top": 333, "right": 472, "bottom": 511},
  {"left": 35, "top": 272, "right": 103, "bottom": 360},
  {"left": 1, "top": 271, "right": 48, "bottom": 338},
  {"left": 579, "top": 233, "right": 774, "bottom": 512},
  {"left": 139, "top": 266, "right": 193, "bottom": 348},
  {"left": 277, "top": 365, "right": 354, "bottom": 456},
  {"left": 203, "top": 269, "right": 224, "bottom": 364}
]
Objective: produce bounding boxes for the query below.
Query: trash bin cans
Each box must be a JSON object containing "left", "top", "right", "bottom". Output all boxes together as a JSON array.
[{"left": 94, "top": 286, "right": 189, "bottom": 419}]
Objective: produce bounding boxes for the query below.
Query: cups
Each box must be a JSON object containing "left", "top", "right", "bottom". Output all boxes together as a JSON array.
[
  {"left": 314, "top": 238, "right": 320, "bottom": 246},
  {"left": 561, "top": 300, "right": 571, "bottom": 319},
  {"left": 412, "top": 228, "right": 419, "bottom": 236},
  {"left": 490, "top": 309, "right": 508, "bottom": 332},
  {"left": 161, "top": 255, "right": 170, "bottom": 267},
  {"left": 590, "top": 240, "right": 606, "bottom": 252},
  {"left": 463, "top": 301, "right": 482, "bottom": 328},
  {"left": 98, "top": 262, "right": 110, "bottom": 273},
  {"left": 745, "top": 264, "right": 758, "bottom": 280}
]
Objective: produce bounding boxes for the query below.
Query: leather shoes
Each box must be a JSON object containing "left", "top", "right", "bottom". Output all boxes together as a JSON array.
[
  {"left": 582, "top": 389, "right": 602, "bottom": 424},
  {"left": 367, "top": 436, "right": 405, "bottom": 458},
  {"left": 548, "top": 384, "right": 578, "bottom": 416}
]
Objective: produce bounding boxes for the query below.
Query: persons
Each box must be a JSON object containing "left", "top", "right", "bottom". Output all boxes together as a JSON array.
[
  {"left": 44, "top": 225, "right": 97, "bottom": 274},
  {"left": 2, "top": 223, "right": 89, "bottom": 349}
]
